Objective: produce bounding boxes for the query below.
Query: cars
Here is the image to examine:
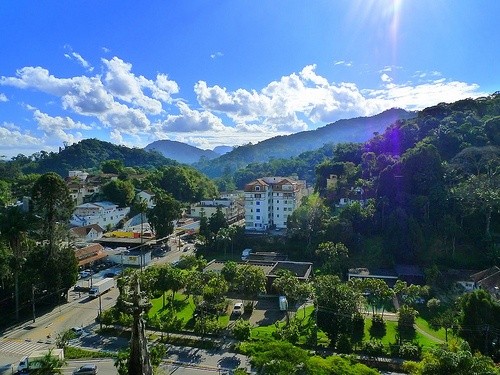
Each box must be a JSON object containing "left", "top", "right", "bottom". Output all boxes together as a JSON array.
[
  {"left": 232, "top": 301, "right": 243, "bottom": 314},
  {"left": 71, "top": 364, "right": 98, "bottom": 375},
  {"left": 69, "top": 327, "right": 85, "bottom": 335},
  {"left": 75, "top": 260, "right": 118, "bottom": 280}
]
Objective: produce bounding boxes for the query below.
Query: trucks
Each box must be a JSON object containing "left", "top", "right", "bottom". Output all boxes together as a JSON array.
[
  {"left": 17, "top": 348, "right": 63, "bottom": 375},
  {"left": 89, "top": 277, "right": 115, "bottom": 298},
  {"left": 241, "top": 248, "right": 252, "bottom": 261},
  {"left": 278, "top": 295, "right": 288, "bottom": 311}
]
